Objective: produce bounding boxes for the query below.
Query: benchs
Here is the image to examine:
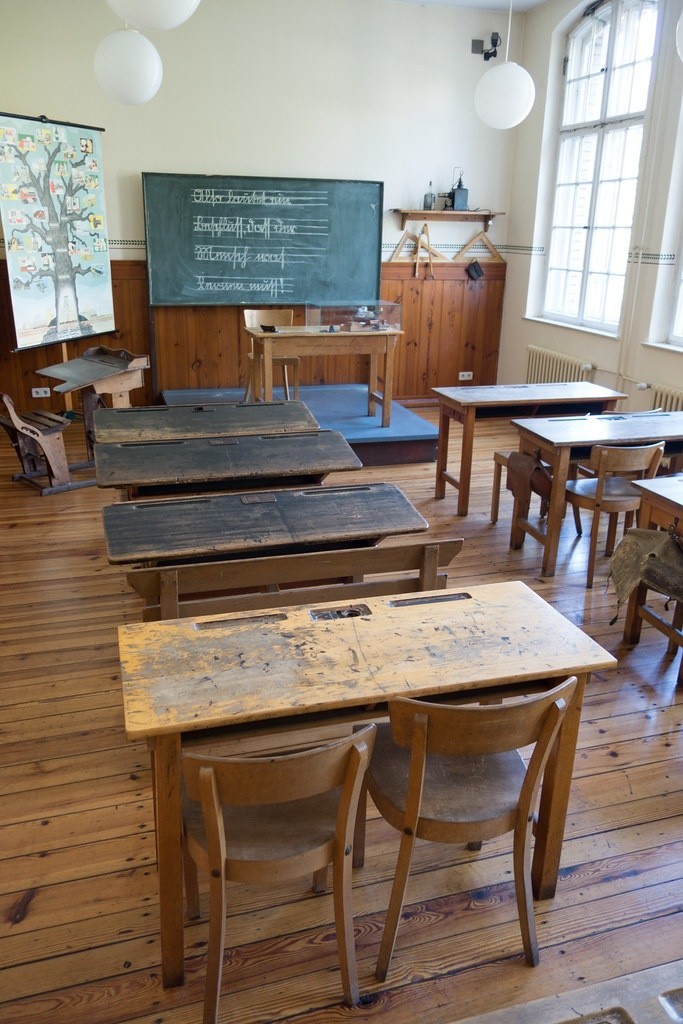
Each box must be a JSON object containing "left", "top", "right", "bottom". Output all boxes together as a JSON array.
[{"left": 126, "top": 538, "right": 463, "bottom": 622}]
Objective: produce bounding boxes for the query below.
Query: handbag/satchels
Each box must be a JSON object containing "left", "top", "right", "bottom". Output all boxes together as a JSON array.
[
  {"left": 466, "top": 258, "right": 484, "bottom": 284},
  {"left": 604, "top": 522, "right": 683, "bottom": 626}
]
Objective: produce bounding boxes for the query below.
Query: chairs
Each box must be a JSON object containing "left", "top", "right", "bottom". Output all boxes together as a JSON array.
[
  {"left": 353, "top": 676, "right": 576, "bottom": 983},
  {"left": 566, "top": 441, "right": 666, "bottom": 588},
  {"left": 0, "top": 393, "right": 97, "bottom": 497},
  {"left": 178, "top": 724, "right": 377, "bottom": 1024},
  {"left": 490, "top": 408, "right": 661, "bottom": 535},
  {"left": 242, "top": 309, "right": 300, "bottom": 405}
]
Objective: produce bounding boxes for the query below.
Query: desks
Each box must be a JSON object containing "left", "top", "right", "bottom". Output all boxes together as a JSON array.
[
  {"left": 91, "top": 399, "right": 431, "bottom": 568},
  {"left": 510, "top": 411, "right": 682, "bottom": 577},
  {"left": 35, "top": 345, "right": 151, "bottom": 462},
  {"left": 117, "top": 580, "right": 618, "bottom": 989},
  {"left": 430, "top": 382, "right": 628, "bottom": 516},
  {"left": 244, "top": 326, "right": 405, "bottom": 427},
  {"left": 622, "top": 477, "right": 683, "bottom": 690}
]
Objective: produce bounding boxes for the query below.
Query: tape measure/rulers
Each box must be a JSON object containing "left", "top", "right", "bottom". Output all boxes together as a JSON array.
[
  {"left": 388, "top": 229, "right": 452, "bottom": 263},
  {"left": 453, "top": 230, "right": 506, "bottom": 262}
]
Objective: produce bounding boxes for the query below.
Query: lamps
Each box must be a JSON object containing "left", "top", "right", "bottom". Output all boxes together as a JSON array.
[
  {"left": 474, "top": 1, "right": 535, "bottom": 129},
  {"left": 92, "top": 1, "right": 201, "bottom": 104}
]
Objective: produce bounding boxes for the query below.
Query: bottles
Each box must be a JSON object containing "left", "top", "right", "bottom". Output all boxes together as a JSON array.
[{"left": 424, "top": 181, "right": 436, "bottom": 211}]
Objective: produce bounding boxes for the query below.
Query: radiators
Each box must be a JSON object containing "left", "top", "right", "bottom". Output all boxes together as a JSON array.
[
  {"left": 526, "top": 344, "right": 593, "bottom": 384},
  {"left": 652, "top": 386, "right": 683, "bottom": 411}
]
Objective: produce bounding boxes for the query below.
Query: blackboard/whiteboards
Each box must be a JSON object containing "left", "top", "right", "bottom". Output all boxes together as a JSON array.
[{"left": 140, "top": 171, "right": 386, "bottom": 307}]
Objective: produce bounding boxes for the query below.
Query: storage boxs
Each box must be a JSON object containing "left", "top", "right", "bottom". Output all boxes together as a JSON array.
[{"left": 306, "top": 301, "right": 401, "bottom": 332}]
